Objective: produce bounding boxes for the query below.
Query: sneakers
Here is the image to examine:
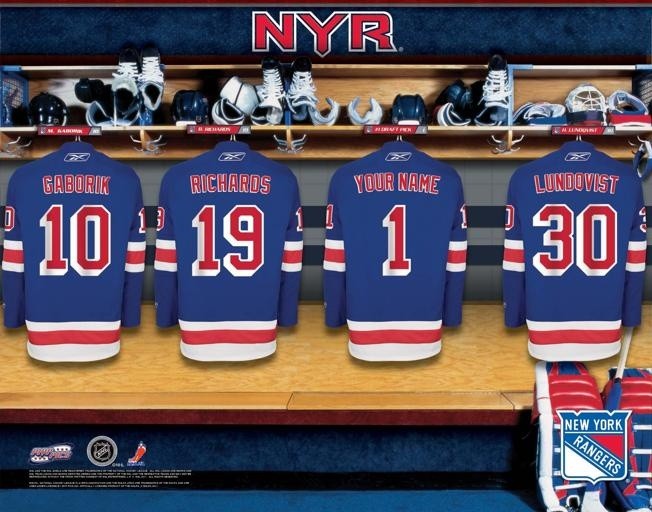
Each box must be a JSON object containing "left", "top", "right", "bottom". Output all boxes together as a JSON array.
[
  {"left": 483, "top": 55, "right": 509, "bottom": 121},
  {"left": 289, "top": 57, "right": 313, "bottom": 121},
  {"left": 112, "top": 46, "right": 140, "bottom": 114},
  {"left": 139, "top": 43, "right": 163, "bottom": 111},
  {"left": 259, "top": 60, "right": 285, "bottom": 123}
]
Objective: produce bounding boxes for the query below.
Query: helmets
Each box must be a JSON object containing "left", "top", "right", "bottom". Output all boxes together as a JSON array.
[
  {"left": 30, "top": 93, "right": 70, "bottom": 127},
  {"left": 391, "top": 94, "right": 426, "bottom": 126},
  {"left": 565, "top": 85, "right": 607, "bottom": 125},
  {"left": 171, "top": 90, "right": 208, "bottom": 123}
]
